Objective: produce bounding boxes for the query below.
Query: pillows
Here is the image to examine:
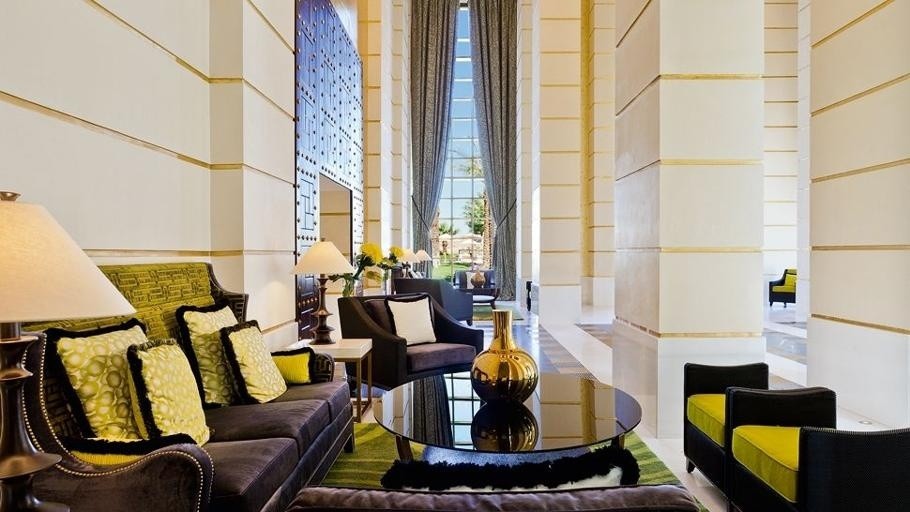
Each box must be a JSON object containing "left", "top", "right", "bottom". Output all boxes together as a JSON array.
[
  {"left": 385, "top": 295, "right": 437, "bottom": 345},
  {"left": 40, "top": 315, "right": 154, "bottom": 443},
  {"left": 59, "top": 433, "right": 196, "bottom": 470},
  {"left": 122, "top": 337, "right": 213, "bottom": 450},
  {"left": 176, "top": 301, "right": 245, "bottom": 406},
  {"left": 216, "top": 319, "right": 286, "bottom": 402}
]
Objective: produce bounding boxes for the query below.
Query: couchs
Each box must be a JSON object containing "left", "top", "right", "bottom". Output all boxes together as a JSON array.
[
  {"left": 770, "top": 266, "right": 797, "bottom": 312},
  {"left": 290, "top": 487, "right": 700, "bottom": 512},
  {"left": 393, "top": 278, "right": 474, "bottom": 326},
  {"left": 0, "top": 261, "right": 356, "bottom": 512},
  {"left": 338, "top": 290, "right": 483, "bottom": 388},
  {"left": 452, "top": 270, "right": 500, "bottom": 310}
]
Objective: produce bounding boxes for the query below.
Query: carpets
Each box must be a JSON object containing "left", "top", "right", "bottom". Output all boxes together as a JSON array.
[{"left": 318, "top": 422, "right": 710, "bottom": 512}]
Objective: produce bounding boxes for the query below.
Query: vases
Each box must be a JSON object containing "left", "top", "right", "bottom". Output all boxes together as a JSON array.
[
  {"left": 470, "top": 309, "right": 539, "bottom": 403},
  {"left": 470, "top": 266, "right": 486, "bottom": 289}
]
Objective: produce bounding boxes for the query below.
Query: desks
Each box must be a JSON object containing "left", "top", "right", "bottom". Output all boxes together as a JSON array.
[{"left": 282, "top": 337, "right": 373, "bottom": 423}]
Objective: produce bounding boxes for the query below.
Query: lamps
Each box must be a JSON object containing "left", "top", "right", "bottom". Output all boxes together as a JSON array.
[
  {"left": 288, "top": 241, "right": 357, "bottom": 344},
  {"left": 1, "top": 190, "right": 137, "bottom": 512},
  {"left": 416, "top": 249, "right": 432, "bottom": 272},
  {"left": 397, "top": 248, "right": 421, "bottom": 278}
]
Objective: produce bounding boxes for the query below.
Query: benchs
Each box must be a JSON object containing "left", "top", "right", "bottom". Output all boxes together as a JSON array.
[
  {"left": 684, "top": 361, "right": 836, "bottom": 492},
  {"left": 730, "top": 388, "right": 909, "bottom": 512}
]
